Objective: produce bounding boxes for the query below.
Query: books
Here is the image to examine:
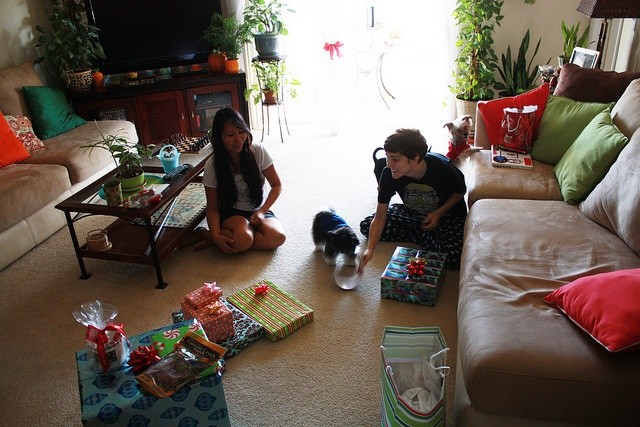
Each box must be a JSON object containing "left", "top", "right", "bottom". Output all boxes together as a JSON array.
[{"left": 491, "top": 144, "right": 533, "bottom": 170}]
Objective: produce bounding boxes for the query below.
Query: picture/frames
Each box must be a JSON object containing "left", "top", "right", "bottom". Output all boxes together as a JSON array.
[{"left": 568, "top": 47, "right": 600, "bottom": 69}]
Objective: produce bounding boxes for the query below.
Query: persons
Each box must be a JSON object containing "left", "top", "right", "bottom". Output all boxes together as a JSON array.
[
  {"left": 176, "top": 106, "right": 286, "bottom": 254},
  {"left": 357, "top": 127, "right": 467, "bottom": 275}
]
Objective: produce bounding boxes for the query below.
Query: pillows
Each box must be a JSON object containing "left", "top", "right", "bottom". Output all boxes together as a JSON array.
[
  {"left": 4, "top": 114, "right": 48, "bottom": 152},
  {"left": 552, "top": 107, "right": 628, "bottom": 205},
  {"left": 611, "top": 79, "right": 640, "bottom": 142},
  {"left": 478, "top": 81, "right": 550, "bottom": 153},
  {"left": 23, "top": 85, "right": 88, "bottom": 141},
  {"left": 0, "top": 108, "right": 31, "bottom": 168},
  {"left": 531, "top": 94, "right": 616, "bottom": 165},
  {"left": 579, "top": 125, "right": 640, "bottom": 256},
  {"left": 554, "top": 63, "right": 640, "bottom": 104},
  {"left": 542, "top": 267, "right": 640, "bottom": 354}
]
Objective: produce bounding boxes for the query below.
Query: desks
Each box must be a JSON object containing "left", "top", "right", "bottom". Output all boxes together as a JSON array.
[{"left": 73, "top": 66, "right": 254, "bottom": 148}]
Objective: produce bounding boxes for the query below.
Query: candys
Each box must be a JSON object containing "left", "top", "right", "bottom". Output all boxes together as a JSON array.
[
  {"left": 153, "top": 341, "right": 165, "bottom": 352},
  {"left": 216, "top": 367, "right": 223, "bottom": 373},
  {"left": 201, "top": 334, "right": 205, "bottom": 339},
  {"left": 163, "top": 330, "right": 181, "bottom": 340},
  {"left": 189, "top": 323, "right": 202, "bottom": 333}
]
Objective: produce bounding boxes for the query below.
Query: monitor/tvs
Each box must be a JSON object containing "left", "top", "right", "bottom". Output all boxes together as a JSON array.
[{"left": 83, "top": 0, "right": 223, "bottom": 75}]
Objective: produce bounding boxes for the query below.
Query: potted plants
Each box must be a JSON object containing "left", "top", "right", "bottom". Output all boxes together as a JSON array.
[
  {"left": 243, "top": 58, "right": 301, "bottom": 104},
  {"left": 446, "top": 0, "right": 504, "bottom": 137},
  {"left": 558, "top": 19, "right": 597, "bottom": 68},
  {"left": 79, "top": 119, "right": 157, "bottom": 188},
  {"left": 200, "top": 11, "right": 259, "bottom": 75},
  {"left": 490, "top": 29, "right": 553, "bottom": 99},
  {"left": 33, "top": 0, "right": 106, "bottom": 100},
  {"left": 238, "top": 0, "right": 296, "bottom": 58}
]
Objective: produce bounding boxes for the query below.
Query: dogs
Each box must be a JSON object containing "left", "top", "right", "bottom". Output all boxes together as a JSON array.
[{"left": 442, "top": 116, "right": 485, "bottom": 168}]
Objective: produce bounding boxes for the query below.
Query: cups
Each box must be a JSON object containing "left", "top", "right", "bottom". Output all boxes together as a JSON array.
[{"left": 334, "top": 259, "right": 361, "bottom": 290}]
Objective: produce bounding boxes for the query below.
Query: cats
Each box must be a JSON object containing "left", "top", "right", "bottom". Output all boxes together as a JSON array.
[{"left": 311, "top": 206, "right": 360, "bottom": 266}]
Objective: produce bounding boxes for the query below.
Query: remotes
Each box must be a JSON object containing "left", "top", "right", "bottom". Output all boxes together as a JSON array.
[{"left": 163, "top": 165, "right": 188, "bottom": 180}]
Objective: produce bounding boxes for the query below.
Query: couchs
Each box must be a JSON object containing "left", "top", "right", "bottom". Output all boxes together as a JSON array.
[
  {"left": 0, "top": 58, "right": 139, "bottom": 271},
  {"left": 454, "top": 99, "right": 640, "bottom": 427}
]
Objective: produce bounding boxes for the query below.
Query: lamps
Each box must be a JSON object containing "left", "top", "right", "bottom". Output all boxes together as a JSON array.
[{"left": 576, "top": 0, "right": 640, "bottom": 72}]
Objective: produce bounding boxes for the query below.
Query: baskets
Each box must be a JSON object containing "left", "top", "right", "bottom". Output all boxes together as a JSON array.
[{"left": 156, "top": 145, "right": 181, "bottom": 175}]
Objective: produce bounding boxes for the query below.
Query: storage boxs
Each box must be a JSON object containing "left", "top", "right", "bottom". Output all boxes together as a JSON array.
[{"left": 75, "top": 319, "right": 231, "bottom": 427}]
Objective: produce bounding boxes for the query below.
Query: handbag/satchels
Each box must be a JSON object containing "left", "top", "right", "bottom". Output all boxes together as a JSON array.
[
  {"left": 382, "top": 326, "right": 450, "bottom": 427},
  {"left": 499, "top": 106, "right": 538, "bottom": 154}
]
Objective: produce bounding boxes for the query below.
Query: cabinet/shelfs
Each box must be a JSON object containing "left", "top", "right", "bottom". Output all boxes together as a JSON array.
[
  {"left": 74, "top": 75, "right": 142, "bottom": 145},
  {"left": 252, "top": 56, "right": 292, "bottom": 143}
]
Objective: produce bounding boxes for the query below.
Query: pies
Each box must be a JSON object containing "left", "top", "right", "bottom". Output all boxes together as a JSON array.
[{"left": 174, "top": 343, "right": 177, "bottom": 348}]
